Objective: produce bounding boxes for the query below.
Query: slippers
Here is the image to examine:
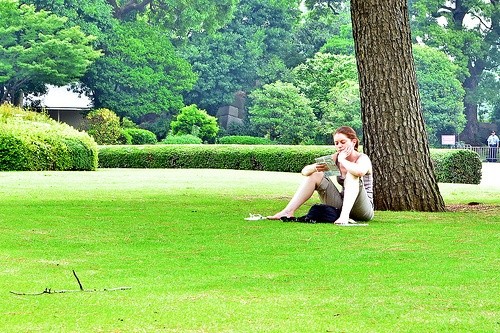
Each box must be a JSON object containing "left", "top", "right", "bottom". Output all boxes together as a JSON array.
[
  {"left": 244, "top": 212, "right": 267, "bottom": 220},
  {"left": 341, "top": 218, "right": 368, "bottom": 227}
]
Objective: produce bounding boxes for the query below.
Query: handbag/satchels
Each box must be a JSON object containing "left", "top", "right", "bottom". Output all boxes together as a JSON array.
[{"left": 306, "top": 204, "right": 340, "bottom": 223}]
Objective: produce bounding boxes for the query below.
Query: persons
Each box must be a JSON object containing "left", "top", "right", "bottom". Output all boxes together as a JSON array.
[
  {"left": 486, "top": 129, "right": 499, "bottom": 161},
  {"left": 267, "top": 125, "right": 375, "bottom": 225}
]
